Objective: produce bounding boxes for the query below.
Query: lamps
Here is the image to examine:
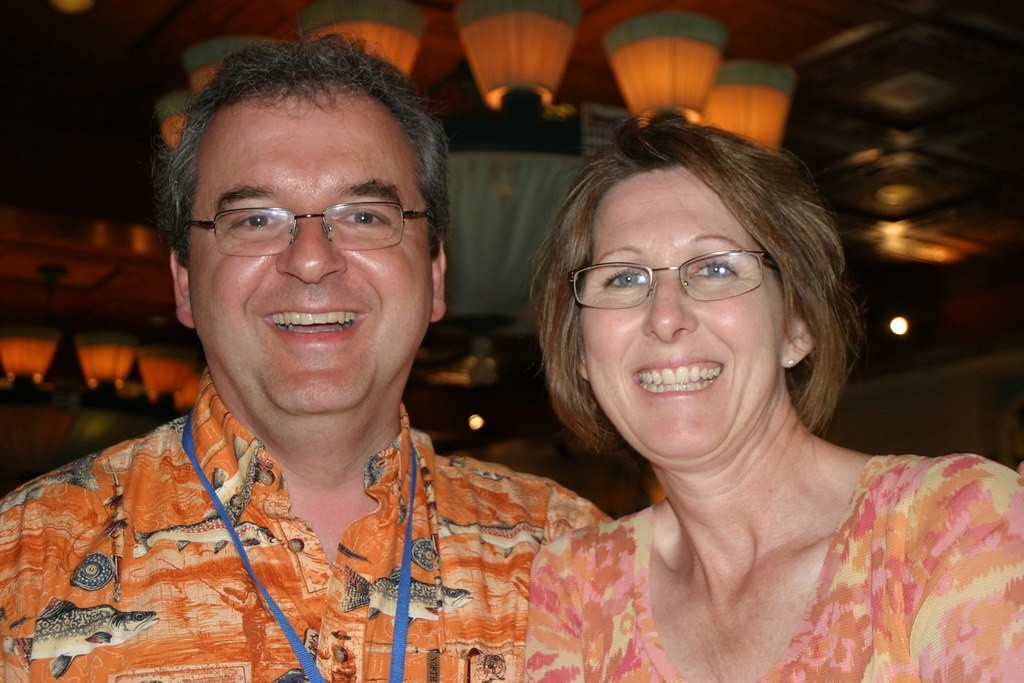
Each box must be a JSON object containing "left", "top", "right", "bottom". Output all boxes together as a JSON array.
[
  {"left": 158, "top": 95, "right": 191, "bottom": 145},
  {"left": 298, "top": 0, "right": 427, "bottom": 76},
  {"left": 181, "top": 36, "right": 274, "bottom": 93},
  {"left": 603, "top": 11, "right": 726, "bottom": 126},
  {"left": 456, "top": 0, "right": 583, "bottom": 110},
  {"left": 706, "top": 59, "right": 798, "bottom": 149}
]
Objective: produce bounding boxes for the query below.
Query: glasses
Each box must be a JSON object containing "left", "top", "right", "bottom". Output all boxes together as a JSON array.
[
  {"left": 569, "top": 251, "right": 779, "bottom": 310},
  {"left": 190, "top": 201, "right": 429, "bottom": 257}
]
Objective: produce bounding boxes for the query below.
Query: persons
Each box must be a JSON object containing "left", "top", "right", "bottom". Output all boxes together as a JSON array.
[
  {"left": 525, "top": 111, "right": 1024, "bottom": 683},
  {"left": 0, "top": 31, "right": 616, "bottom": 683}
]
